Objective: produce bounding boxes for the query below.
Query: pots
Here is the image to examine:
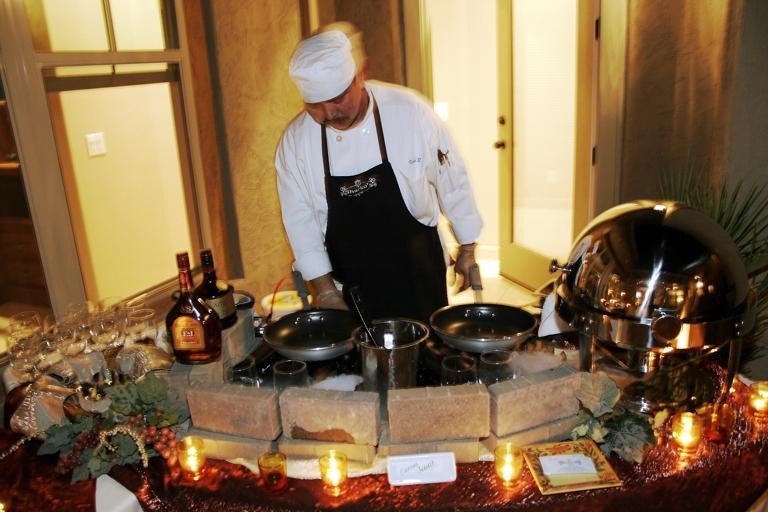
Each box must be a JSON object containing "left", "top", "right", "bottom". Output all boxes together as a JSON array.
[
  {"left": 263, "top": 271, "right": 363, "bottom": 361},
  {"left": 353, "top": 318, "right": 429, "bottom": 388},
  {"left": 430, "top": 263, "right": 538, "bottom": 353}
]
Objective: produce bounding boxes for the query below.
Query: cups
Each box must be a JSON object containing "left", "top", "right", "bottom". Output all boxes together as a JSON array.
[
  {"left": 229, "top": 356, "right": 260, "bottom": 388},
  {"left": 258, "top": 451, "right": 289, "bottom": 496},
  {"left": 747, "top": 382, "right": 768, "bottom": 416},
  {"left": 479, "top": 350, "right": 515, "bottom": 384},
  {"left": 440, "top": 356, "right": 476, "bottom": 385},
  {"left": 272, "top": 360, "right": 310, "bottom": 390},
  {"left": 672, "top": 412, "right": 703, "bottom": 457},
  {"left": 319, "top": 449, "right": 350, "bottom": 497},
  {"left": 176, "top": 435, "right": 208, "bottom": 480},
  {"left": 494, "top": 442, "right": 523, "bottom": 493}
]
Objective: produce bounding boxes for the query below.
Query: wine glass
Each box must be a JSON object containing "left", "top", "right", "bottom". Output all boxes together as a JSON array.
[{"left": 5, "top": 296, "right": 159, "bottom": 393}]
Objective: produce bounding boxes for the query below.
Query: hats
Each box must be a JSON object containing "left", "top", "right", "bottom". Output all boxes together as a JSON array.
[{"left": 289, "top": 31, "right": 356, "bottom": 104}]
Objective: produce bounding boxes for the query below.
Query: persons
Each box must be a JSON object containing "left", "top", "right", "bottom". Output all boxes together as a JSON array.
[{"left": 275, "top": 22, "right": 486, "bottom": 326}]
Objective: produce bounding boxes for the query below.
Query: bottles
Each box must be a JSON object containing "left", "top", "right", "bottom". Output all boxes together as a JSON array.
[
  {"left": 164, "top": 253, "right": 221, "bottom": 364},
  {"left": 194, "top": 249, "right": 237, "bottom": 329}
]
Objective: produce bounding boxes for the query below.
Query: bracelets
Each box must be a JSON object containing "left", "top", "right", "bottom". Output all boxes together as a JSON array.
[
  {"left": 315, "top": 289, "right": 340, "bottom": 303},
  {"left": 462, "top": 249, "right": 473, "bottom": 256}
]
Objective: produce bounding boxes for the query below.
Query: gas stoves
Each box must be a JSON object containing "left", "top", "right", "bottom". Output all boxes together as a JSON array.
[{"left": 231, "top": 342, "right": 533, "bottom": 390}]
